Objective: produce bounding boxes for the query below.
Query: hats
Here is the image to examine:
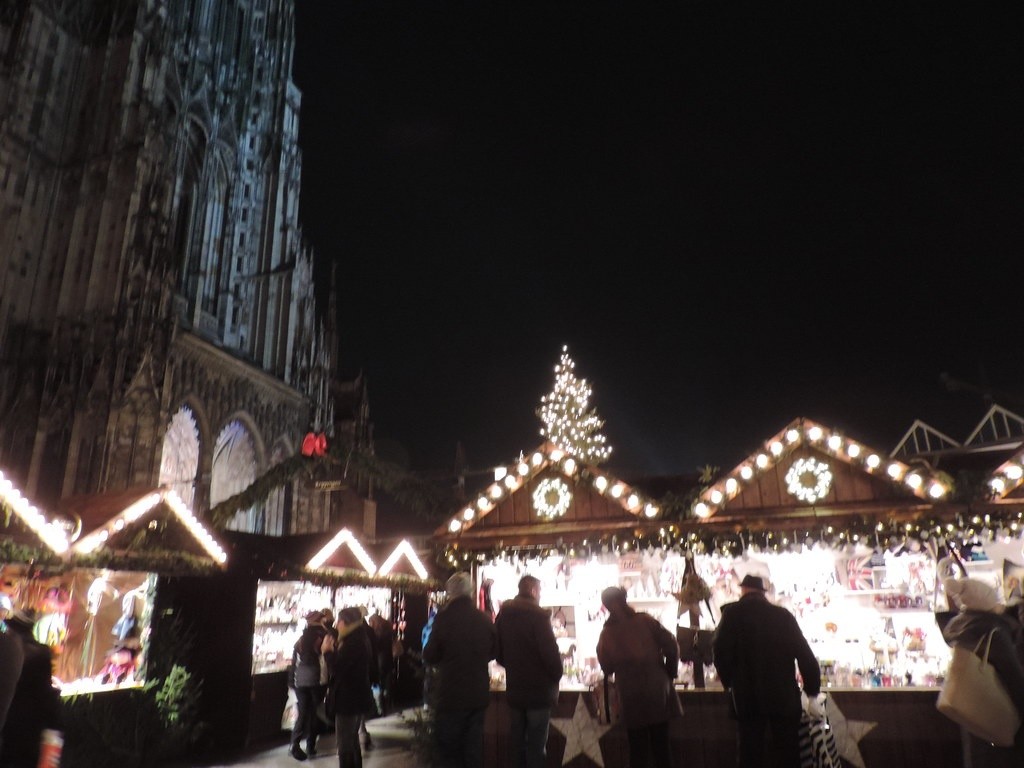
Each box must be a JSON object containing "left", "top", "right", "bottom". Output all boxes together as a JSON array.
[
  {"left": 447, "top": 572, "right": 474, "bottom": 595},
  {"left": 739, "top": 575, "right": 767, "bottom": 591},
  {"left": 945, "top": 579, "right": 996, "bottom": 611},
  {"left": 305, "top": 612, "right": 324, "bottom": 624}
]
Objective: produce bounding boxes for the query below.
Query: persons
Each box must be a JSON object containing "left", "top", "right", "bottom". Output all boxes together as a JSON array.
[
  {"left": 943, "top": 577, "right": 1024, "bottom": 768},
  {"left": 598, "top": 588, "right": 678, "bottom": 768},
  {"left": 493, "top": 575, "right": 563, "bottom": 768},
  {"left": 0, "top": 608, "right": 64, "bottom": 768},
  {"left": 713, "top": 576, "right": 821, "bottom": 767},
  {"left": 288, "top": 609, "right": 394, "bottom": 768},
  {"left": 422, "top": 573, "right": 494, "bottom": 768}
]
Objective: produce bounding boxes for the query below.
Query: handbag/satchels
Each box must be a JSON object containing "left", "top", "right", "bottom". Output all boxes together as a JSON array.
[
  {"left": 936, "top": 625, "right": 1020, "bottom": 747},
  {"left": 391, "top": 631, "right": 404, "bottom": 658},
  {"left": 678, "top": 627, "right": 717, "bottom": 666}
]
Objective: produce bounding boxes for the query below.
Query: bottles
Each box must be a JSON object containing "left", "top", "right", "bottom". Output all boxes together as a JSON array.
[
  {"left": 819, "top": 661, "right": 911, "bottom": 687},
  {"left": 562, "top": 656, "right": 599, "bottom": 686}
]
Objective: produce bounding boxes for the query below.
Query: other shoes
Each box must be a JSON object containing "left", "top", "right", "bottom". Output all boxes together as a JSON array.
[
  {"left": 290, "top": 744, "right": 307, "bottom": 761},
  {"left": 306, "top": 747, "right": 317, "bottom": 755}
]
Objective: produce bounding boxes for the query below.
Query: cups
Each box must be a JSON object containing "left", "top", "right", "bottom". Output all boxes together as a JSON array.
[
  {"left": 37, "top": 735, "right": 64, "bottom": 768},
  {"left": 876, "top": 595, "right": 922, "bottom": 608}
]
